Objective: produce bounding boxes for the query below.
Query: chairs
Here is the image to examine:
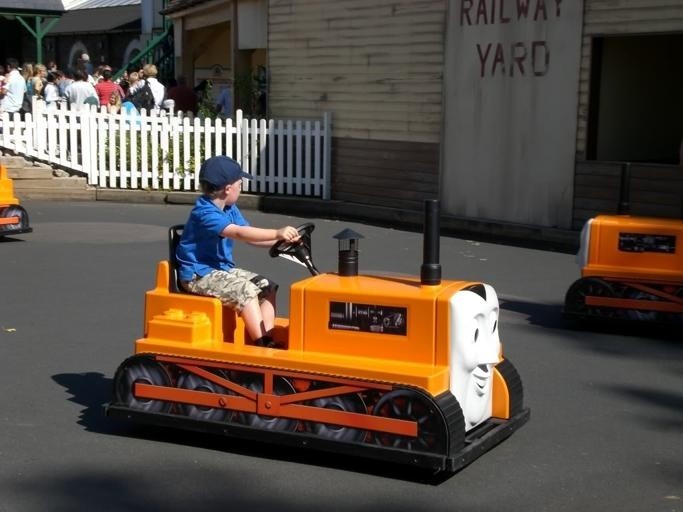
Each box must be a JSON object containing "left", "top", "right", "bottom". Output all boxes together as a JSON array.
[{"left": 167, "top": 225, "right": 188, "bottom": 295}]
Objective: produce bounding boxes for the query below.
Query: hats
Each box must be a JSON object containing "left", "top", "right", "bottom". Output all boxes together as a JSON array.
[{"left": 199, "top": 155, "right": 253, "bottom": 190}]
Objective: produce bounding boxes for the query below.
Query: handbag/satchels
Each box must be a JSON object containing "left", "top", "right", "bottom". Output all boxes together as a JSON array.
[
  {"left": 110, "top": 86, "right": 122, "bottom": 110},
  {"left": 129, "top": 80, "right": 155, "bottom": 113}
]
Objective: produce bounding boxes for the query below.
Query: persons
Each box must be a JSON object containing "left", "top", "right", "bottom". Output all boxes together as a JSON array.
[
  {"left": 175, "top": 154, "right": 299, "bottom": 347},
  {"left": 214, "top": 79, "right": 234, "bottom": 121},
  {"left": 0, "top": 51, "right": 199, "bottom": 123}
]
